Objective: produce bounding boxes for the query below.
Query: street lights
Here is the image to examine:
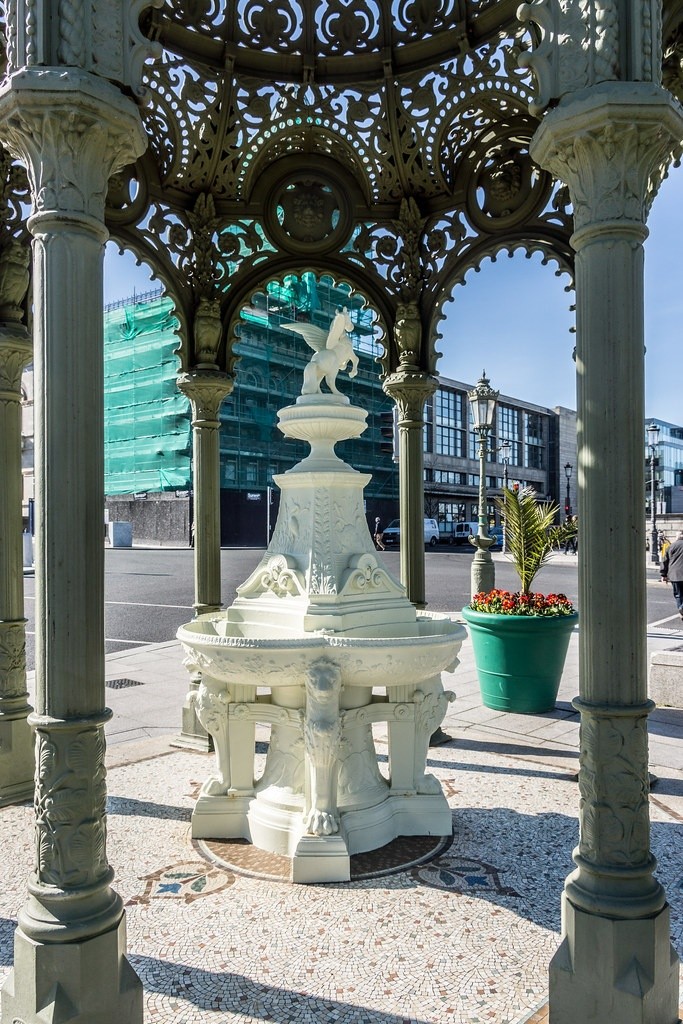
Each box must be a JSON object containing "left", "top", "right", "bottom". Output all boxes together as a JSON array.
[
  {"left": 466, "top": 368, "right": 502, "bottom": 607},
  {"left": 564, "top": 462, "right": 573, "bottom": 515},
  {"left": 500, "top": 439, "right": 511, "bottom": 554},
  {"left": 646, "top": 421, "right": 661, "bottom": 563}
]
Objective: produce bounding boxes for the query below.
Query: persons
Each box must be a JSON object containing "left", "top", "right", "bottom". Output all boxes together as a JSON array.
[
  {"left": 23, "top": 527, "right": 29, "bottom": 533},
  {"left": 563, "top": 515, "right": 578, "bottom": 555},
  {"left": 374, "top": 517, "right": 386, "bottom": 551},
  {"left": 660, "top": 530, "right": 683, "bottom": 620},
  {"left": 190, "top": 523, "right": 194, "bottom": 548}
]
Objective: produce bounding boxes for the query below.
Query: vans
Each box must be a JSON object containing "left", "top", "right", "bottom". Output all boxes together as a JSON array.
[
  {"left": 453, "top": 521, "right": 479, "bottom": 546},
  {"left": 381, "top": 518, "right": 441, "bottom": 547}
]
